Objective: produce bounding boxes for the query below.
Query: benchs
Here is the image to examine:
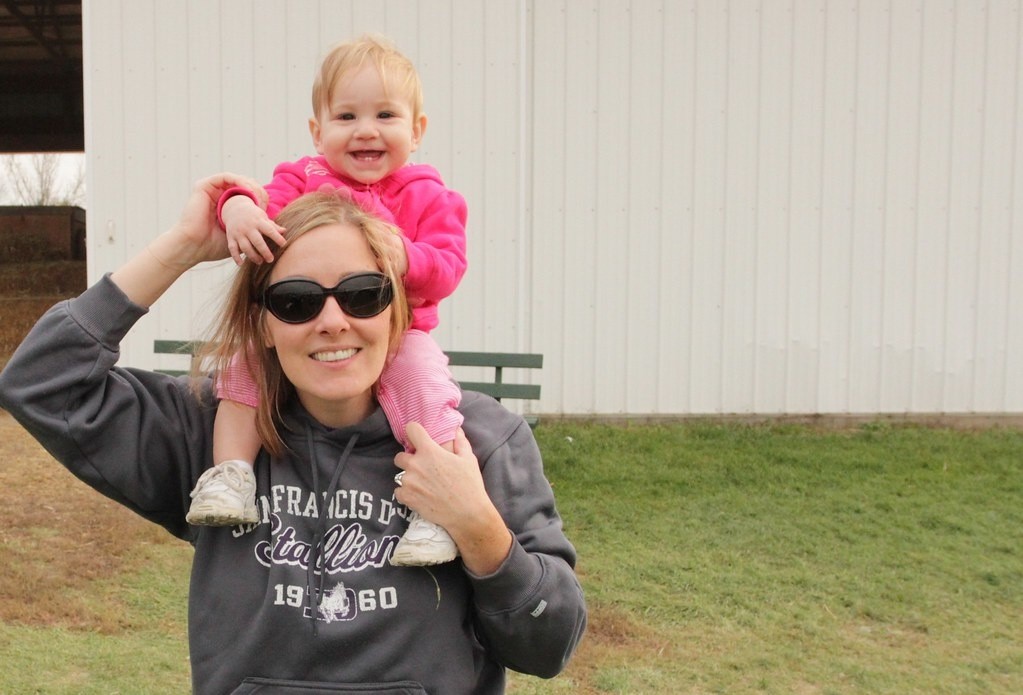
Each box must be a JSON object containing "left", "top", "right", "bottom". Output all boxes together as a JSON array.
[{"left": 154, "top": 340, "right": 546, "bottom": 428}]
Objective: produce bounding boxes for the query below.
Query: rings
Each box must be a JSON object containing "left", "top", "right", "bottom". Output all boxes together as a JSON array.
[{"left": 394, "top": 469, "right": 406, "bottom": 486}]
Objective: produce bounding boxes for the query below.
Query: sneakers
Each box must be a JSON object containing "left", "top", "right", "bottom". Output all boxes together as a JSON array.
[
  {"left": 391, "top": 512, "right": 460, "bottom": 568},
  {"left": 186, "top": 460, "right": 259, "bottom": 526}
]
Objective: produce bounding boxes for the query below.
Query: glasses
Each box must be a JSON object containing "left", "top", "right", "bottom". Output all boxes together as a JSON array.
[{"left": 249, "top": 271, "right": 394, "bottom": 325}]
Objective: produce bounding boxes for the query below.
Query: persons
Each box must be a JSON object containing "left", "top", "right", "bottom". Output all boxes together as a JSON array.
[
  {"left": 183, "top": 35, "right": 470, "bottom": 565},
  {"left": 0, "top": 175, "right": 588, "bottom": 695}
]
